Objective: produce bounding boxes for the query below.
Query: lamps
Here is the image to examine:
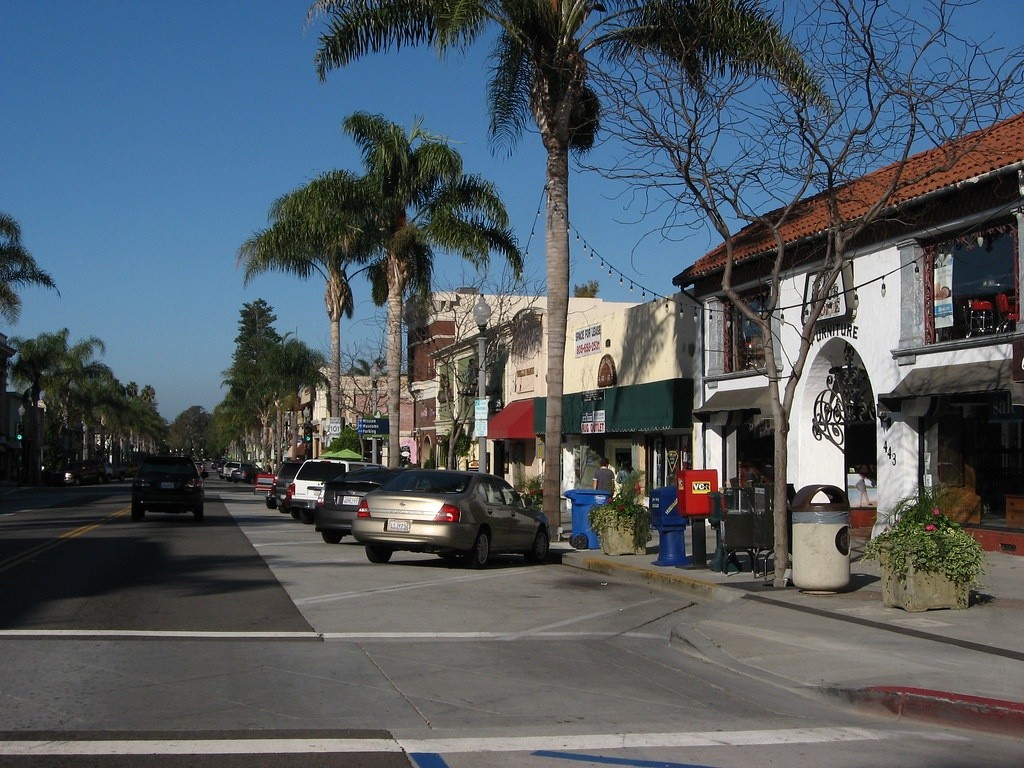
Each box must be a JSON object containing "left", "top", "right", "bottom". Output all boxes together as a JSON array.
[{"left": 812, "top": 340, "right": 891, "bottom": 434}]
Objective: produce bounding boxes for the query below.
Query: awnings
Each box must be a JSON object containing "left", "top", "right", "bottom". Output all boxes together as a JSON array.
[
  {"left": 485, "top": 377, "right": 694, "bottom": 439},
  {"left": 879, "top": 357, "right": 1024, "bottom": 413},
  {"left": 692, "top": 386, "right": 775, "bottom": 426}
]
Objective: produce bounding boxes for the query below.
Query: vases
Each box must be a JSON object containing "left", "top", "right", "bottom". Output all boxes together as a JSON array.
[
  {"left": 878, "top": 552, "right": 969, "bottom": 611},
  {"left": 600, "top": 519, "right": 647, "bottom": 555}
]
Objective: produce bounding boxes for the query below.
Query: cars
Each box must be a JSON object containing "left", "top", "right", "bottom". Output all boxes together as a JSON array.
[
  {"left": 131, "top": 453, "right": 210, "bottom": 520},
  {"left": 232, "top": 467, "right": 263, "bottom": 484},
  {"left": 45, "top": 459, "right": 129, "bottom": 487},
  {"left": 313, "top": 466, "right": 421, "bottom": 546},
  {"left": 210, "top": 456, "right": 253, "bottom": 479},
  {"left": 351, "top": 468, "right": 551, "bottom": 568},
  {"left": 195, "top": 457, "right": 207, "bottom": 476}
]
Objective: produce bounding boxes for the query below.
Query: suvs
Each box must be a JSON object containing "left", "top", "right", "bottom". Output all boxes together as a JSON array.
[
  {"left": 284, "top": 459, "right": 386, "bottom": 526},
  {"left": 263, "top": 458, "right": 303, "bottom": 514}
]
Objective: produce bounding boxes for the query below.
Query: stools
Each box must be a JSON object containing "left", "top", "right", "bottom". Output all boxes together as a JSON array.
[{"left": 965, "top": 301, "right": 995, "bottom": 338}]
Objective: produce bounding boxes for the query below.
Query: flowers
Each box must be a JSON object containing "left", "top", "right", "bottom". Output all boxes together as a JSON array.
[
  {"left": 860, "top": 482, "right": 994, "bottom": 605},
  {"left": 587, "top": 470, "right": 656, "bottom": 554}
]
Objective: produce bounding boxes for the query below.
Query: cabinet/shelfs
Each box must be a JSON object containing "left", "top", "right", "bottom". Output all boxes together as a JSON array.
[{"left": 1003, "top": 495, "right": 1024, "bottom": 528}]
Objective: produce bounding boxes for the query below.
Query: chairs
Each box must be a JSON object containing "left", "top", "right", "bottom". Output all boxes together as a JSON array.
[
  {"left": 439, "top": 477, "right": 462, "bottom": 495},
  {"left": 995, "top": 294, "right": 1016, "bottom": 333}
]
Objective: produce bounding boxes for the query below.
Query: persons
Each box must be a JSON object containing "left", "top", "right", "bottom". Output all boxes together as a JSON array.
[
  {"left": 593, "top": 457, "right": 639, "bottom": 496},
  {"left": 933, "top": 281, "right": 951, "bottom": 300}
]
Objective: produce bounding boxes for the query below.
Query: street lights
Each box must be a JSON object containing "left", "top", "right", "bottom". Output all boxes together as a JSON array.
[{"left": 473, "top": 293, "right": 493, "bottom": 473}]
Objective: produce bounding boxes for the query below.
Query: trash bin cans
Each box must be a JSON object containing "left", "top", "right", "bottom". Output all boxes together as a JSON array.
[
  {"left": 789, "top": 484, "right": 853, "bottom": 593},
  {"left": 721, "top": 488, "right": 752, "bottom": 549},
  {"left": 563, "top": 489, "right": 610, "bottom": 548},
  {"left": 753, "top": 484, "right": 795, "bottom": 549}
]
